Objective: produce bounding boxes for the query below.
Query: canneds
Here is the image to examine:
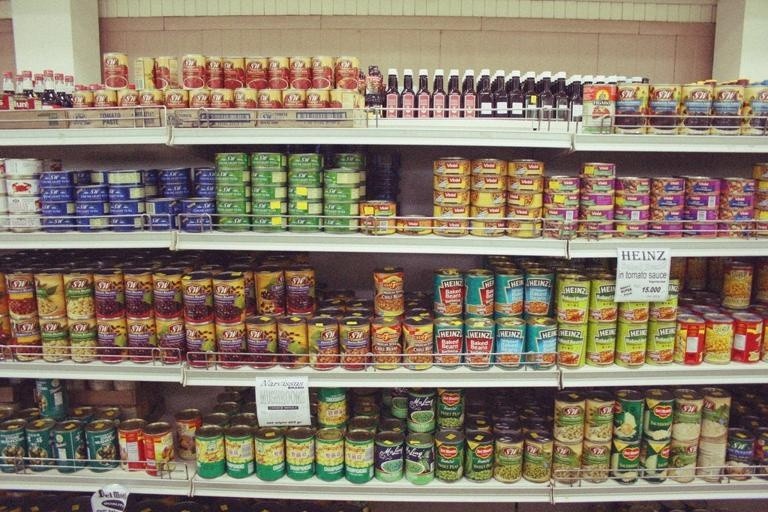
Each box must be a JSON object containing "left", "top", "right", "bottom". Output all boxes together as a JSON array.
[
  {"left": 196, "top": 385, "right": 768, "bottom": 484},
  {"left": 433, "top": 156, "right": 768, "bottom": 240},
  {"left": 649, "top": 84, "right": 682, "bottom": 134},
  {"left": 357, "top": 199, "right": 432, "bottom": 236},
  {"left": 42, "top": 166, "right": 216, "bottom": 233},
  {"left": 679, "top": 84, "right": 713, "bottom": 135},
  {"left": 174, "top": 408, "right": 202, "bottom": 460},
  {"left": 1, "top": 254, "right": 767, "bottom": 376},
  {"left": 215, "top": 149, "right": 368, "bottom": 233},
  {"left": 582, "top": 83, "right": 616, "bottom": 134},
  {"left": 742, "top": 85, "right": 767, "bottom": 136},
  {"left": 72, "top": 52, "right": 365, "bottom": 108},
  {"left": 710, "top": 85, "right": 745, "bottom": 135},
  {"left": 1, "top": 379, "right": 174, "bottom": 476},
  {"left": 617, "top": 83, "right": 649, "bottom": 134},
  {"left": 0, "top": 154, "right": 62, "bottom": 232}
]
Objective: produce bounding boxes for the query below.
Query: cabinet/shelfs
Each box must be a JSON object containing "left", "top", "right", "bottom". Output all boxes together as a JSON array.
[{"left": 0, "top": 108, "right": 767, "bottom": 505}]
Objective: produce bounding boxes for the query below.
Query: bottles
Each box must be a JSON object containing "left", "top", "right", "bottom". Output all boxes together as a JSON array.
[
  {"left": 359, "top": 64, "right": 651, "bottom": 122},
  {"left": 2, "top": 69, "right": 75, "bottom": 110}
]
